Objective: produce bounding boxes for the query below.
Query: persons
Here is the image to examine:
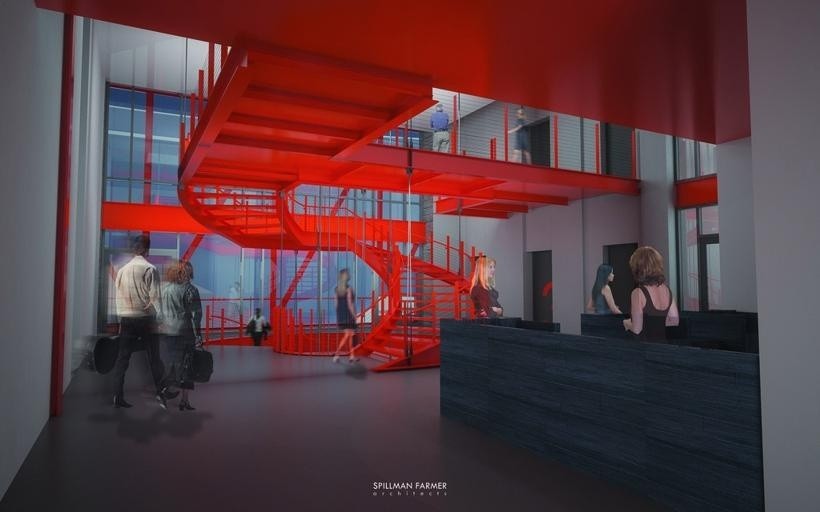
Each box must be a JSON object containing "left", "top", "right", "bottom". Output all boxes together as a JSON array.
[
  {"left": 246, "top": 306, "right": 267, "bottom": 346},
  {"left": 509, "top": 104, "right": 533, "bottom": 165},
  {"left": 429, "top": 103, "right": 451, "bottom": 155},
  {"left": 176, "top": 261, "right": 205, "bottom": 351},
  {"left": 110, "top": 233, "right": 182, "bottom": 408},
  {"left": 585, "top": 264, "right": 622, "bottom": 314},
  {"left": 468, "top": 254, "right": 504, "bottom": 320},
  {"left": 330, "top": 267, "right": 361, "bottom": 364},
  {"left": 622, "top": 244, "right": 679, "bottom": 340},
  {"left": 153, "top": 259, "right": 197, "bottom": 413}
]
